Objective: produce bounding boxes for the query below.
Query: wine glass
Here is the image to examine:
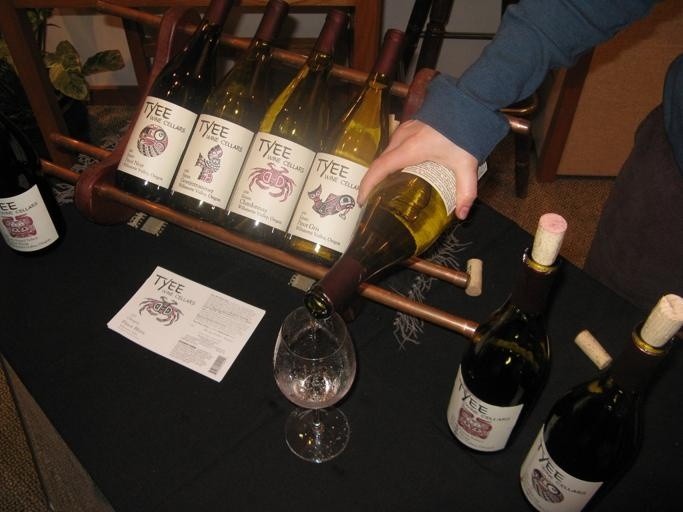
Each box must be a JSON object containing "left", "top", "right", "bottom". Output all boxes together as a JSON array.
[{"left": 273, "top": 306, "right": 358, "bottom": 464}]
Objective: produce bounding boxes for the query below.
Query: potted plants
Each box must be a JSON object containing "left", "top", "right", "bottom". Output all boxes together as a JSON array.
[{"left": 1, "top": 5, "right": 126, "bottom": 160}]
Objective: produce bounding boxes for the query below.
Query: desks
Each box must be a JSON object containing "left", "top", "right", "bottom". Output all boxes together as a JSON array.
[{"left": 1, "top": 199, "right": 683, "bottom": 512}]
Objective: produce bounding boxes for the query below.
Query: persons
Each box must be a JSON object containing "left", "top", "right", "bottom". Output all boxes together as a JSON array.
[{"left": 353, "top": 0, "right": 683, "bottom": 314}]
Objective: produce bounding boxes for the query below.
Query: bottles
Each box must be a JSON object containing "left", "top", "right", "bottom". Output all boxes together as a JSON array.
[
  {"left": 171, "top": 3, "right": 292, "bottom": 219},
  {"left": 108, "top": 0, "right": 240, "bottom": 193},
  {"left": 0, "top": 108, "right": 65, "bottom": 258},
  {"left": 285, "top": 31, "right": 437, "bottom": 266},
  {"left": 224, "top": 12, "right": 357, "bottom": 246},
  {"left": 516, "top": 290, "right": 683, "bottom": 512},
  {"left": 445, "top": 209, "right": 570, "bottom": 461},
  {"left": 308, "top": 150, "right": 497, "bottom": 316}
]
